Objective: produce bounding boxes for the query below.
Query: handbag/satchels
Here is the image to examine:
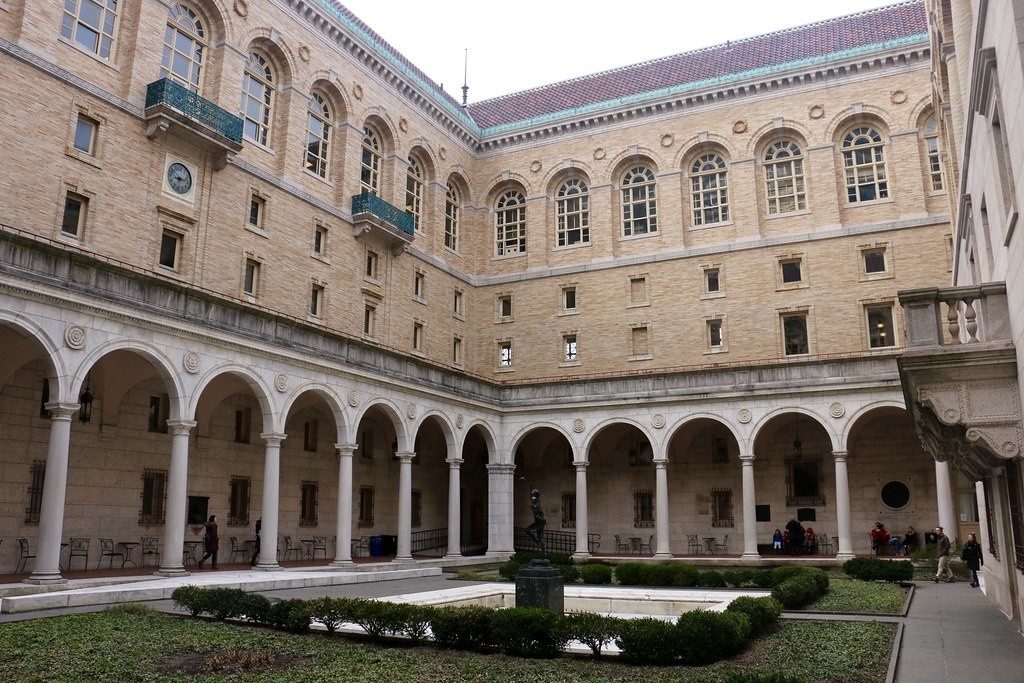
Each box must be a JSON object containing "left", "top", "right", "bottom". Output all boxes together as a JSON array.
[{"left": 960, "top": 550, "right": 968, "bottom": 562}]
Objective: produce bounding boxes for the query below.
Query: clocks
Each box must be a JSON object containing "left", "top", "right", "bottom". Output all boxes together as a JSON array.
[{"left": 168, "top": 162, "right": 193, "bottom": 194}]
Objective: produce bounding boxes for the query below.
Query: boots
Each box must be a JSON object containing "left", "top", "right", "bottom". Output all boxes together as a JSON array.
[
  {"left": 211, "top": 563, "right": 219, "bottom": 570},
  {"left": 198, "top": 558, "right": 205, "bottom": 570},
  {"left": 250, "top": 559, "right": 256, "bottom": 566}
]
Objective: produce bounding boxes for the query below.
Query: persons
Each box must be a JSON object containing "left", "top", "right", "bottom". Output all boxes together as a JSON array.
[
  {"left": 250, "top": 517, "right": 262, "bottom": 566},
  {"left": 782, "top": 516, "right": 815, "bottom": 555},
  {"left": 773, "top": 529, "right": 783, "bottom": 554},
  {"left": 520, "top": 477, "right": 547, "bottom": 560},
  {"left": 871, "top": 522, "right": 901, "bottom": 558},
  {"left": 899, "top": 526, "right": 920, "bottom": 557},
  {"left": 198, "top": 515, "right": 219, "bottom": 570},
  {"left": 964, "top": 533, "right": 983, "bottom": 587},
  {"left": 930, "top": 527, "right": 954, "bottom": 583}
]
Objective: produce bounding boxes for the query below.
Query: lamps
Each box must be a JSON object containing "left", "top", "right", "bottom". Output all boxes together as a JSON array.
[
  {"left": 629, "top": 433, "right": 637, "bottom": 468},
  {"left": 392, "top": 438, "right": 400, "bottom": 462},
  {"left": 793, "top": 414, "right": 802, "bottom": 459},
  {"left": 78, "top": 370, "right": 93, "bottom": 425}
]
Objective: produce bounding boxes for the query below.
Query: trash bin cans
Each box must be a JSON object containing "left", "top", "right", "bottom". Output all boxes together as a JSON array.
[{"left": 370, "top": 535, "right": 396, "bottom": 556}]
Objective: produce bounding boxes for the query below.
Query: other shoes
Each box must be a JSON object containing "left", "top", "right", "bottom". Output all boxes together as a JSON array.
[
  {"left": 944, "top": 577, "right": 955, "bottom": 583},
  {"left": 871, "top": 544, "right": 877, "bottom": 550},
  {"left": 970, "top": 581, "right": 975, "bottom": 588},
  {"left": 930, "top": 576, "right": 939, "bottom": 583},
  {"left": 975, "top": 582, "right": 979, "bottom": 586},
  {"left": 876, "top": 551, "right": 880, "bottom": 556}
]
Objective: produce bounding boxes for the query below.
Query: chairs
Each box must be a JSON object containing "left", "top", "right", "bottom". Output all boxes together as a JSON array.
[
  {"left": 687, "top": 535, "right": 702, "bottom": 555},
  {"left": 68, "top": 538, "right": 91, "bottom": 571},
  {"left": 615, "top": 535, "right": 629, "bottom": 554},
  {"left": 15, "top": 539, "right": 36, "bottom": 573},
  {"left": 277, "top": 537, "right": 281, "bottom": 562},
  {"left": 284, "top": 535, "right": 302, "bottom": 565},
  {"left": 97, "top": 538, "right": 124, "bottom": 569},
  {"left": 360, "top": 536, "right": 373, "bottom": 557},
  {"left": 229, "top": 537, "right": 249, "bottom": 563},
  {"left": 141, "top": 537, "right": 160, "bottom": 568},
  {"left": 313, "top": 536, "right": 328, "bottom": 559},
  {"left": 641, "top": 535, "right": 654, "bottom": 555},
  {"left": 816, "top": 534, "right": 833, "bottom": 556},
  {"left": 715, "top": 535, "right": 729, "bottom": 555},
  {"left": 868, "top": 533, "right": 921, "bottom": 555}
]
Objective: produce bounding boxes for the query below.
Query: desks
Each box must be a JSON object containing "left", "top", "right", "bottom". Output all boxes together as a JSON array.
[
  {"left": 118, "top": 542, "right": 139, "bottom": 568},
  {"left": 184, "top": 541, "right": 203, "bottom": 567},
  {"left": 702, "top": 538, "right": 715, "bottom": 555},
  {"left": 832, "top": 537, "right": 839, "bottom": 553},
  {"left": 300, "top": 540, "right": 317, "bottom": 560},
  {"left": 351, "top": 539, "right": 360, "bottom": 558},
  {"left": 628, "top": 538, "right": 641, "bottom": 554},
  {"left": 244, "top": 539, "right": 260, "bottom": 563},
  {"left": 60, "top": 543, "right": 68, "bottom": 573}
]
